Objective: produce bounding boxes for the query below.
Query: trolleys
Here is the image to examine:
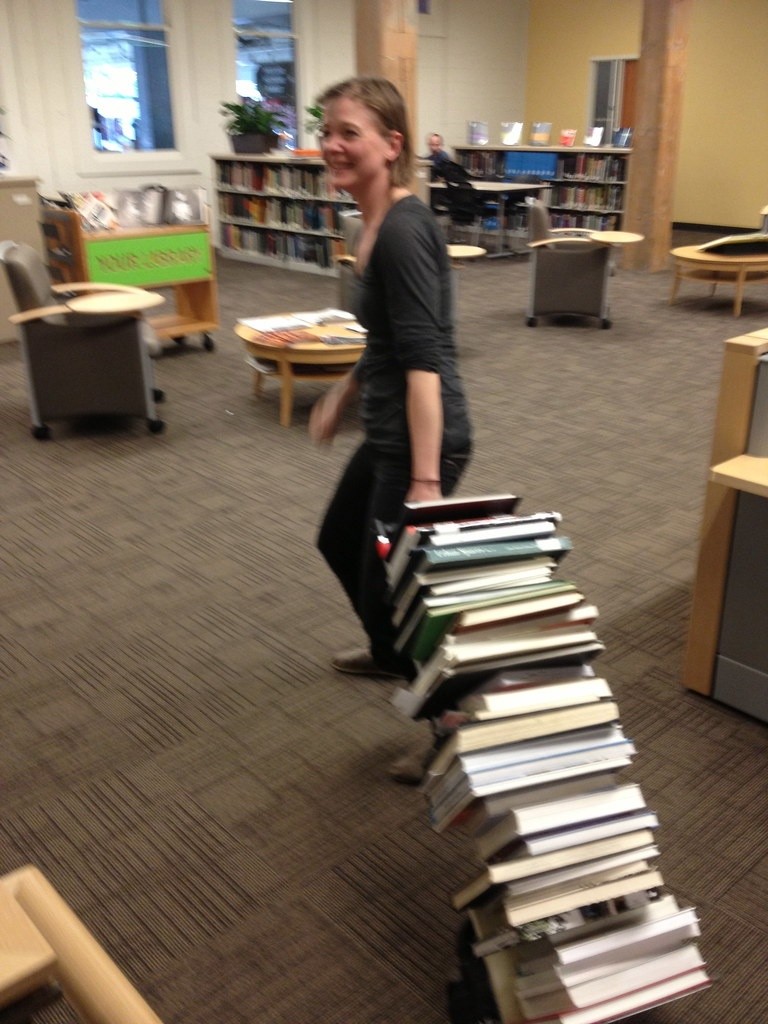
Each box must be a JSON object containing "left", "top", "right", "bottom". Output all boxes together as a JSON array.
[{"left": 369, "top": 508, "right": 666, "bottom": 1024}]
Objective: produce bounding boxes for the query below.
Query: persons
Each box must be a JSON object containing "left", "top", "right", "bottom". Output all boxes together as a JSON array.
[
  {"left": 307, "top": 78, "right": 476, "bottom": 788},
  {"left": 428, "top": 136, "right": 450, "bottom": 168}
]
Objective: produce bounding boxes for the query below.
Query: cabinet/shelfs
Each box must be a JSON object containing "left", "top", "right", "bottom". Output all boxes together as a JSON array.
[
  {"left": 208, "top": 151, "right": 358, "bottom": 279},
  {"left": 685, "top": 326, "right": 768, "bottom": 718},
  {"left": 0, "top": 175, "right": 54, "bottom": 342},
  {"left": 450, "top": 146, "right": 630, "bottom": 242},
  {"left": 44, "top": 210, "right": 221, "bottom": 355}
]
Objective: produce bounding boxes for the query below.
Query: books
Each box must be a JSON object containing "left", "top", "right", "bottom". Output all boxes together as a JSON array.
[
  {"left": 459, "top": 120, "right": 637, "bottom": 243},
  {"left": 59, "top": 184, "right": 208, "bottom": 235},
  {"left": 380, "top": 494, "right": 716, "bottom": 1024},
  {"left": 238, "top": 307, "right": 370, "bottom": 375},
  {"left": 211, "top": 158, "right": 358, "bottom": 271}
]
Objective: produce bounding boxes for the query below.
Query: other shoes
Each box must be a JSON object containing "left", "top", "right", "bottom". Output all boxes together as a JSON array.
[
  {"left": 390, "top": 756, "right": 431, "bottom": 786},
  {"left": 332, "top": 647, "right": 403, "bottom": 679}
]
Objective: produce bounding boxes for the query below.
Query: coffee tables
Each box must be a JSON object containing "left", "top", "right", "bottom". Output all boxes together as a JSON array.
[{"left": 237, "top": 312, "right": 367, "bottom": 426}]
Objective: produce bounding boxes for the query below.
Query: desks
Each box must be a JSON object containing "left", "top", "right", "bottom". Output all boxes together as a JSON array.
[
  {"left": 428, "top": 178, "right": 552, "bottom": 261},
  {"left": 444, "top": 245, "right": 484, "bottom": 260},
  {"left": 589, "top": 230, "right": 644, "bottom": 245},
  {"left": 66, "top": 289, "right": 166, "bottom": 313},
  {"left": 669, "top": 245, "right": 768, "bottom": 317}
]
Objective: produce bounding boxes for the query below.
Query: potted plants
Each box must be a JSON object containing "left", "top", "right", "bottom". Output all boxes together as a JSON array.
[{"left": 218, "top": 101, "right": 287, "bottom": 155}]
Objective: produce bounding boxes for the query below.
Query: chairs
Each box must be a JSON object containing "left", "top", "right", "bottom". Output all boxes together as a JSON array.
[
  {"left": 0, "top": 242, "right": 168, "bottom": 439},
  {"left": 527, "top": 198, "right": 614, "bottom": 330}
]
